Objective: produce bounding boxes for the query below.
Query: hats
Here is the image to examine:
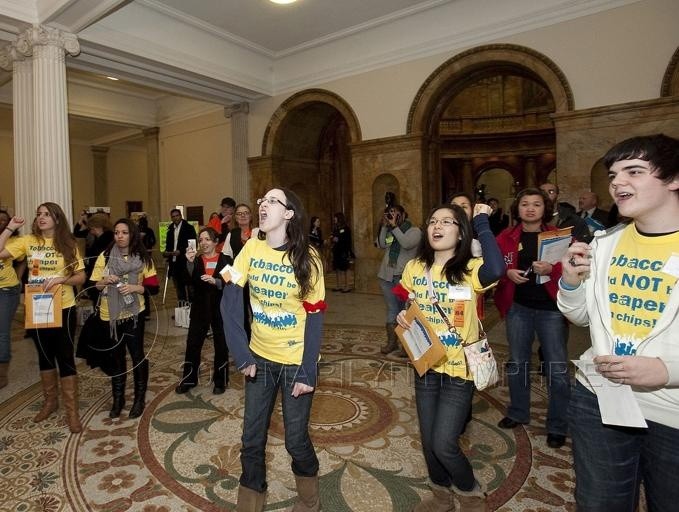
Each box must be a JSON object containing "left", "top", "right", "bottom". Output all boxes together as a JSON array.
[{"left": 221, "top": 197, "right": 235, "bottom": 207}]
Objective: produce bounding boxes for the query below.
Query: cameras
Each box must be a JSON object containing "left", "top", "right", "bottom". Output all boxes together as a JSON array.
[{"left": 384, "top": 208, "right": 395, "bottom": 220}]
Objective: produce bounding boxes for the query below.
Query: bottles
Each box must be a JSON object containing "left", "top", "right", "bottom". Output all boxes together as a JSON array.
[
  {"left": 116, "top": 280, "right": 134, "bottom": 304},
  {"left": 405, "top": 293, "right": 420, "bottom": 317}
]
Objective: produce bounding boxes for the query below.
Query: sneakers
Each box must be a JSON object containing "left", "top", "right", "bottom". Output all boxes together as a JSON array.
[
  {"left": 498, "top": 417, "right": 518, "bottom": 428},
  {"left": 547, "top": 434, "right": 564, "bottom": 446}
]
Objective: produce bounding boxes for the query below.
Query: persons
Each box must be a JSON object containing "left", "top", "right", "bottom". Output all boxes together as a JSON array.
[
  {"left": 374, "top": 133, "right": 679, "bottom": 448},
  {"left": 555, "top": 133, "right": 678, "bottom": 511},
  {"left": 327, "top": 208, "right": 356, "bottom": 294},
  {"left": 390, "top": 204, "right": 507, "bottom": 512},
  {"left": 308, "top": 215, "right": 327, "bottom": 251},
  {"left": 220, "top": 185, "right": 327, "bottom": 511},
  {"left": 1, "top": 197, "right": 252, "bottom": 435}
]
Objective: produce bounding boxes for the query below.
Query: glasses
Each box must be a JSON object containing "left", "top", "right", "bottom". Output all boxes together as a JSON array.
[
  {"left": 427, "top": 217, "right": 458, "bottom": 227},
  {"left": 257, "top": 197, "right": 290, "bottom": 208}
]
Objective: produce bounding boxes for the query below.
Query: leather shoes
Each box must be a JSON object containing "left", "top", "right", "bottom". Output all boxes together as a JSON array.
[
  {"left": 341, "top": 289, "right": 351, "bottom": 293},
  {"left": 332, "top": 288, "right": 342, "bottom": 291}
]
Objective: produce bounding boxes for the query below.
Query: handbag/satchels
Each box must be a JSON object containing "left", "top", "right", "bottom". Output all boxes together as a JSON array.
[
  {"left": 174, "top": 300, "right": 191, "bottom": 328},
  {"left": 464, "top": 336, "right": 500, "bottom": 390}
]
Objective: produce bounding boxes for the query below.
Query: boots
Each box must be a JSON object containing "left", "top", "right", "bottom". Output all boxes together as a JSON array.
[
  {"left": 380, "top": 323, "right": 397, "bottom": 353},
  {"left": 452, "top": 481, "right": 490, "bottom": 512},
  {"left": 413, "top": 479, "right": 456, "bottom": 511},
  {"left": 398, "top": 346, "right": 407, "bottom": 356},
  {"left": 292, "top": 475, "right": 321, "bottom": 511},
  {"left": 1, "top": 360, "right": 227, "bottom": 433}
]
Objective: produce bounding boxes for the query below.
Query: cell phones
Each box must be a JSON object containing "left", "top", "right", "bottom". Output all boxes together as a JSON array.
[{"left": 188, "top": 239, "right": 197, "bottom": 251}]
